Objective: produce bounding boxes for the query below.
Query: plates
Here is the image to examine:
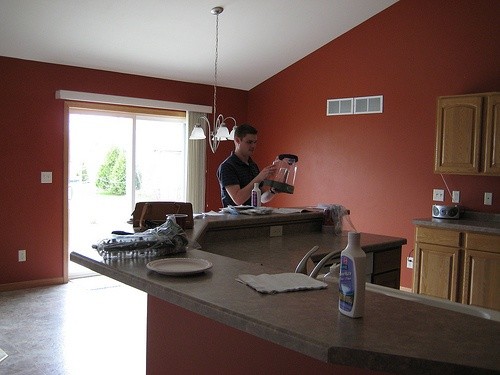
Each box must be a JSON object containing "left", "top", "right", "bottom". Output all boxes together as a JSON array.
[{"left": 145, "top": 258, "right": 213, "bottom": 275}]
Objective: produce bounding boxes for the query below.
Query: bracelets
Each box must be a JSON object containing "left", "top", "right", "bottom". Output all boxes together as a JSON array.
[{"left": 269, "top": 188, "right": 275, "bottom": 194}]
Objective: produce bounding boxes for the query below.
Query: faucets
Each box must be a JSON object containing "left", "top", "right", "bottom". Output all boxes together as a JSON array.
[
  {"left": 293, "top": 244, "right": 322, "bottom": 274},
  {"left": 308, "top": 248, "right": 344, "bottom": 279}
]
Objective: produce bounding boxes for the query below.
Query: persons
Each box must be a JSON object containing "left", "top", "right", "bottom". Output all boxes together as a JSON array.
[{"left": 216, "top": 124, "right": 287, "bottom": 208}]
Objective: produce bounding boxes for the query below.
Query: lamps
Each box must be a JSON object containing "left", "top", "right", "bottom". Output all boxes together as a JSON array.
[{"left": 189, "top": 7, "right": 237, "bottom": 153}]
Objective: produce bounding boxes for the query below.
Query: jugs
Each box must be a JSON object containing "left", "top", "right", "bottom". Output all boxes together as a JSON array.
[{"left": 263, "top": 154, "right": 299, "bottom": 194}]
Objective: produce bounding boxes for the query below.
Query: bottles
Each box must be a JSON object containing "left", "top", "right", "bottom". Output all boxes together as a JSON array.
[
  {"left": 339, "top": 231, "right": 367, "bottom": 318},
  {"left": 251, "top": 183, "right": 261, "bottom": 207}
]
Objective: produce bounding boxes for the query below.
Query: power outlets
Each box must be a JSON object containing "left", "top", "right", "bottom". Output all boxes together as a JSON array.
[
  {"left": 269, "top": 225, "right": 283, "bottom": 237},
  {"left": 484, "top": 193, "right": 492, "bottom": 205},
  {"left": 451, "top": 190, "right": 459, "bottom": 204},
  {"left": 433, "top": 189, "right": 444, "bottom": 202},
  {"left": 406, "top": 256, "right": 413, "bottom": 268}
]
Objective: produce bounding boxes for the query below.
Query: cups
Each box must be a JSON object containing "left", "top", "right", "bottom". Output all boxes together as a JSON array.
[{"left": 166, "top": 213, "right": 188, "bottom": 231}]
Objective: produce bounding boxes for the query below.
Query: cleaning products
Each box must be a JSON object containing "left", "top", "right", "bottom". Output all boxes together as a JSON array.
[{"left": 338, "top": 230, "right": 367, "bottom": 318}]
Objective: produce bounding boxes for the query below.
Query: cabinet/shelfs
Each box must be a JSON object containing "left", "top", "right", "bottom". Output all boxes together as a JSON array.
[
  {"left": 412, "top": 224, "right": 500, "bottom": 313},
  {"left": 434, "top": 92, "right": 500, "bottom": 177}
]
